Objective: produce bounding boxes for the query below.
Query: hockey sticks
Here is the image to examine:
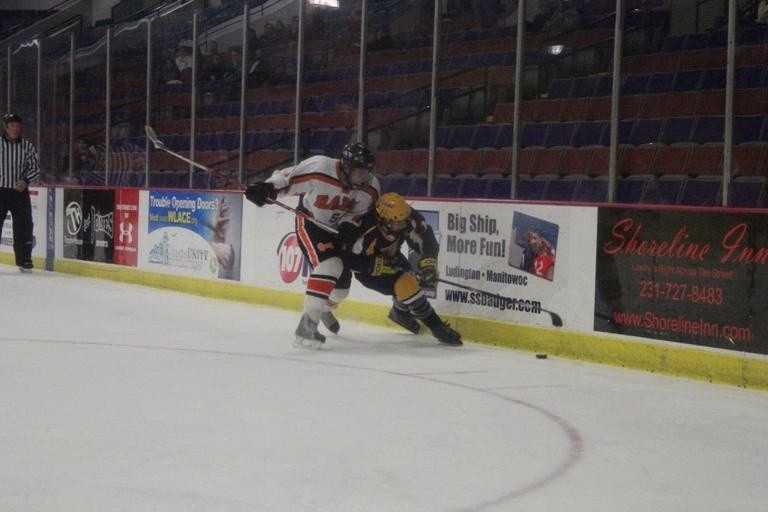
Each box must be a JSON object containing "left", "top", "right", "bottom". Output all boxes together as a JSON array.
[
  {"left": 145, "top": 126, "right": 339, "bottom": 235},
  {"left": 387, "top": 264, "right": 562, "bottom": 327}
]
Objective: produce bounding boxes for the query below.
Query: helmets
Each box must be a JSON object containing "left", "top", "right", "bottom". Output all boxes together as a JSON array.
[
  {"left": 3, "top": 114, "right": 22, "bottom": 123},
  {"left": 340, "top": 143, "right": 375, "bottom": 189},
  {"left": 375, "top": 192, "right": 412, "bottom": 233}
]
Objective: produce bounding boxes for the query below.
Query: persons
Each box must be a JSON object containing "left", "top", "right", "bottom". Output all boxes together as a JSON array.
[
  {"left": 78, "top": 113, "right": 149, "bottom": 262},
  {"left": 244, "top": 141, "right": 380, "bottom": 349},
  {"left": 155, "top": 0, "right": 583, "bottom": 107},
  {"left": 0, "top": 114, "right": 39, "bottom": 269},
  {"left": 190, "top": 197, "right": 235, "bottom": 272},
  {"left": 338, "top": 192, "right": 464, "bottom": 346},
  {"left": 513, "top": 226, "right": 555, "bottom": 280}
]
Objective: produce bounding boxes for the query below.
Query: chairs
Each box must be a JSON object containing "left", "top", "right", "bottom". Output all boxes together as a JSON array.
[{"left": 5, "top": 2, "right": 765, "bottom": 210}]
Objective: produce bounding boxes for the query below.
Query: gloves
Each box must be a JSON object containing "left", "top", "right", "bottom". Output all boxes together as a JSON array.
[
  {"left": 417, "top": 266, "right": 438, "bottom": 288},
  {"left": 338, "top": 222, "right": 358, "bottom": 243},
  {"left": 244, "top": 181, "right": 277, "bottom": 206},
  {"left": 383, "top": 255, "right": 410, "bottom": 274}
]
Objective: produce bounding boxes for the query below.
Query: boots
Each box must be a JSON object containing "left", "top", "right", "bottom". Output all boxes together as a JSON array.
[
  {"left": 16, "top": 260, "right": 33, "bottom": 268},
  {"left": 295, "top": 312, "right": 340, "bottom": 343},
  {"left": 390, "top": 306, "right": 420, "bottom": 331},
  {"left": 433, "top": 321, "right": 460, "bottom": 341}
]
